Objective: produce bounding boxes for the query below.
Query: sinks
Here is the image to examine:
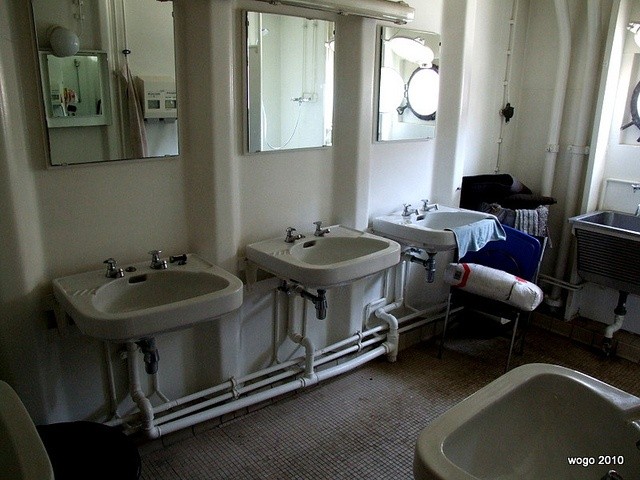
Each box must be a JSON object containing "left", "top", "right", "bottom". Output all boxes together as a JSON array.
[
  {"left": 567, "top": 209, "right": 640, "bottom": 236},
  {"left": 413, "top": 362, "right": 640, "bottom": 480},
  {"left": 52, "top": 253, "right": 243, "bottom": 345},
  {"left": 247, "top": 224, "right": 402, "bottom": 290},
  {"left": 372, "top": 204, "right": 498, "bottom": 253}
]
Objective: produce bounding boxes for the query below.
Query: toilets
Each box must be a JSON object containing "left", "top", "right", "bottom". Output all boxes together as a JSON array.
[{"left": 0, "top": 379, "right": 142, "bottom": 480}]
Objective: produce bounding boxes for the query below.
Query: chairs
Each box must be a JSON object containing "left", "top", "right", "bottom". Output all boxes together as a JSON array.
[{"left": 437, "top": 225, "right": 548, "bottom": 374}]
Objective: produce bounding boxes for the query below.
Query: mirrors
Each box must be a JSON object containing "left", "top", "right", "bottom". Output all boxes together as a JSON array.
[
  {"left": 372, "top": 24, "right": 441, "bottom": 144},
  {"left": 27, "top": 1, "right": 184, "bottom": 170},
  {"left": 384, "top": 36, "right": 434, "bottom": 69},
  {"left": 620, "top": 81, "right": 640, "bottom": 143},
  {"left": 38, "top": 48, "right": 113, "bottom": 129},
  {"left": 618, "top": 53, "right": 640, "bottom": 147},
  {"left": 241, "top": 9, "right": 337, "bottom": 155},
  {"left": 397, "top": 64, "right": 439, "bottom": 121}
]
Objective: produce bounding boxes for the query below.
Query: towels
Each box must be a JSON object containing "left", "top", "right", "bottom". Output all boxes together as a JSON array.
[
  {"left": 444, "top": 215, "right": 506, "bottom": 265},
  {"left": 536, "top": 204, "right": 565, "bottom": 249},
  {"left": 119, "top": 62, "right": 148, "bottom": 159},
  {"left": 515, "top": 209, "right": 538, "bottom": 236}
]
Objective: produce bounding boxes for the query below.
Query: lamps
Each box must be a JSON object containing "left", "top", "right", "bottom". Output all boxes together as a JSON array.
[
  {"left": 47, "top": 23, "right": 80, "bottom": 57},
  {"left": 378, "top": 67, "right": 405, "bottom": 114}
]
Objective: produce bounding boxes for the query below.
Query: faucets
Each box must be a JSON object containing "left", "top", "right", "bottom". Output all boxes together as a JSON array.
[
  {"left": 313, "top": 221, "right": 330, "bottom": 236},
  {"left": 148, "top": 250, "right": 168, "bottom": 269},
  {"left": 632, "top": 185, "right": 640, "bottom": 217},
  {"left": 103, "top": 257, "right": 125, "bottom": 278},
  {"left": 421, "top": 199, "right": 439, "bottom": 211},
  {"left": 402, "top": 203, "right": 419, "bottom": 216},
  {"left": 285, "top": 227, "right": 306, "bottom": 242}
]
